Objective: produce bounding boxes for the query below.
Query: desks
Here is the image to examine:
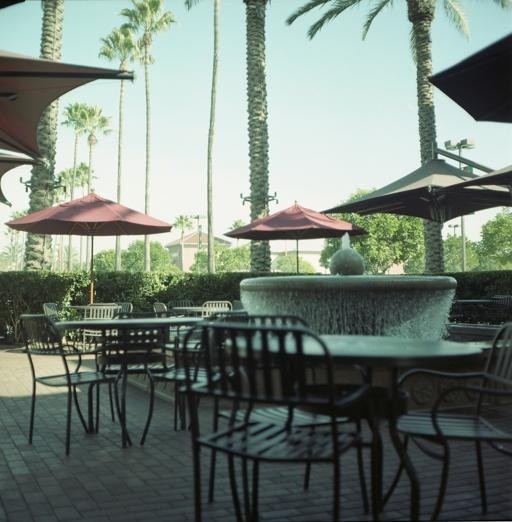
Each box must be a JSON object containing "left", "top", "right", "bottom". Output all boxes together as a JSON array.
[
  {"left": 224, "top": 332, "right": 484, "bottom": 522},
  {"left": 60, "top": 317, "right": 202, "bottom": 443}
]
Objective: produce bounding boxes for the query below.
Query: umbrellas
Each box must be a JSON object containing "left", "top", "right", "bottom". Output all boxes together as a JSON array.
[
  {"left": 1, "top": 48, "right": 132, "bottom": 211},
  {"left": 3, "top": 192, "right": 175, "bottom": 303},
  {"left": 422, "top": 32, "right": 511, "bottom": 128},
  {"left": 314, "top": 146, "right": 511, "bottom": 230},
  {"left": 219, "top": 200, "right": 370, "bottom": 273}
]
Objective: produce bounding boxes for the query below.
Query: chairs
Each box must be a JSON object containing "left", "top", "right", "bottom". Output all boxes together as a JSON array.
[
  {"left": 218, "top": 316, "right": 361, "bottom": 492},
  {"left": 20, "top": 298, "right": 234, "bottom": 370},
  {"left": 141, "top": 309, "right": 246, "bottom": 441},
  {"left": 184, "top": 323, "right": 369, "bottom": 522},
  {"left": 367, "top": 320, "right": 509, "bottom": 522},
  {"left": 20, "top": 313, "right": 126, "bottom": 459}
]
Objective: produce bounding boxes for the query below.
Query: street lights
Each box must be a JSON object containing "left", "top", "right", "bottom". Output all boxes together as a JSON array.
[
  {"left": 189, "top": 213, "right": 206, "bottom": 272},
  {"left": 448, "top": 224, "right": 459, "bottom": 237},
  {"left": 443, "top": 136, "right": 477, "bottom": 273},
  {"left": 85, "top": 130, "right": 98, "bottom": 273}
]
